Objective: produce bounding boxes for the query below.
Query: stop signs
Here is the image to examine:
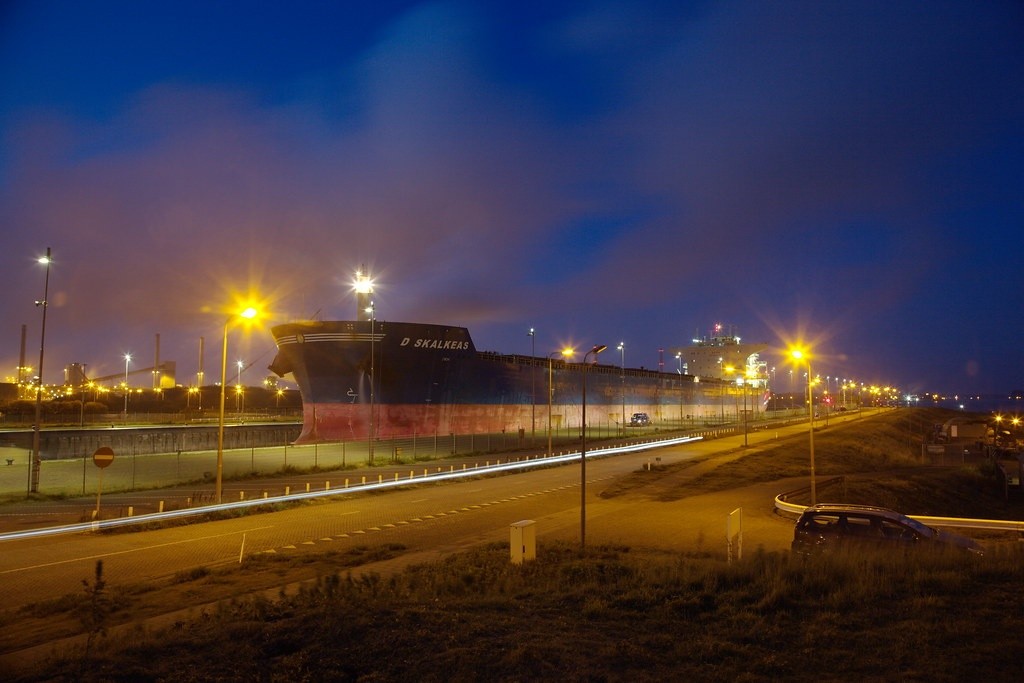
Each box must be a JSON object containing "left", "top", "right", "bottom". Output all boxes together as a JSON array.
[{"left": 91, "top": 446, "right": 116, "bottom": 469}]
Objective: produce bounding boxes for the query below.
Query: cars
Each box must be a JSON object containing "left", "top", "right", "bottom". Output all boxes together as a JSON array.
[
  {"left": 630, "top": 413, "right": 651, "bottom": 426},
  {"left": 788, "top": 499, "right": 986, "bottom": 583}
]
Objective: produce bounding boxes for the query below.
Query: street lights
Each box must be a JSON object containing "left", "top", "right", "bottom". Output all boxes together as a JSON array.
[
  {"left": 364, "top": 301, "right": 377, "bottom": 466},
  {"left": 237, "top": 386, "right": 242, "bottom": 413},
  {"left": 546, "top": 348, "right": 574, "bottom": 457},
  {"left": 187, "top": 389, "right": 194, "bottom": 409},
  {"left": 579, "top": 344, "right": 608, "bottom": 553},
  {"left": 124, "top": 354, "right": 131, "bottom": 425},
  {"left": 30, "top": 244, "right": 54, "bottom": 493},
  {"left": 729, "top": 376, "right": 750, "bottom": 448},
  {"left": 789, "top": 349, "right": 817, "bottom": 505},
  {"left": 234, "top": 360, "right": 244, "bottom": 407},
  {"left": 277, "top": 390, "right": 283, "bottom": 414},
  {"left": 79, "top": 361, "right": 88, "bottom": 428},
  {"left": 716, "top": 357, "right": 725, "bottom": 422},
  {"left": 527, "top": 328, "right": 536, "bottom": 440},
  {"left": 616, "top": 341, "right": 627, "bottom": 435},
  {"left": 772, "top": 366, "right": 900, "bottom": 415},
  {"left": 215, "top": 301, "right": 262, "bottom": 504},
  {"left": 675, "top": 352, "right": 684, "bottom": 425}
]
